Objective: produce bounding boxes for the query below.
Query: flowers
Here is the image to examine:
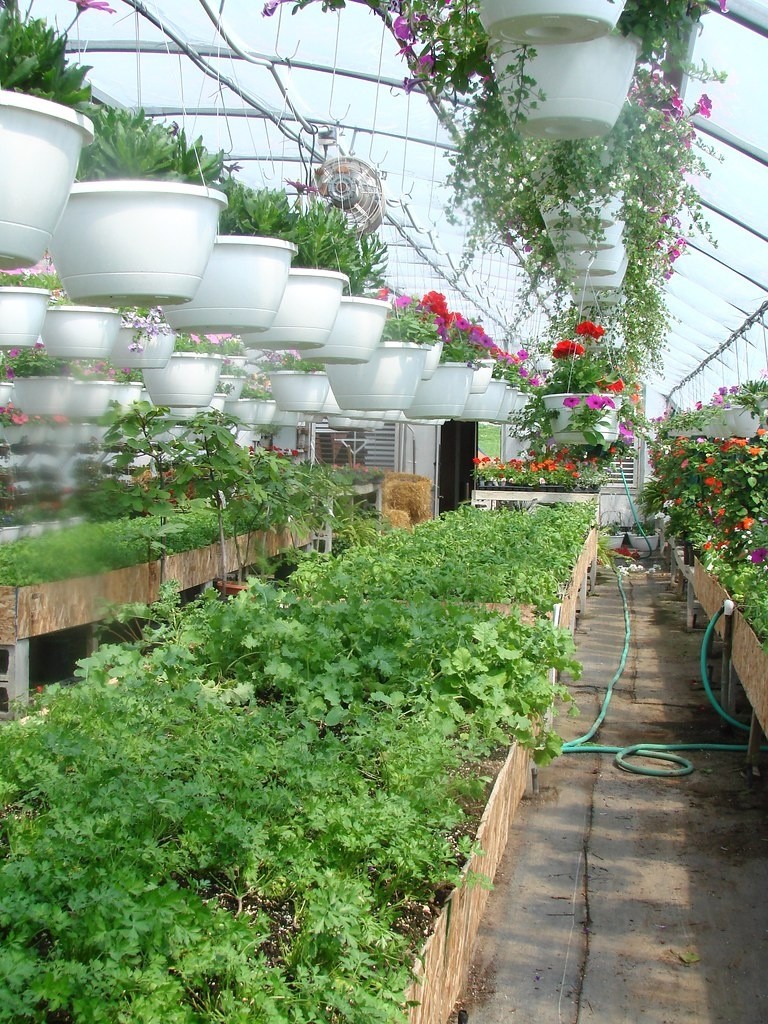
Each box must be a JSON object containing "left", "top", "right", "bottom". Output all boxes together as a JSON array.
[
  {"left": 0, "top": 1, "right": 115, "bottom": 122},
  {"left": 0, "top": 252, "right": 74, "bottom": 306},
  {"left": 329, "top": 287, "right": 644, "bottom": 486},
  {"left": 122, "top": 304, "right": 171, "bottom": 353},
  {"left": 76, "top": 358, "right": 144, "bottom": 385},
  {"left": 5, "top": 341, "right": 73, "bottom": 380},
  {"left": 241, "top": 375, "right": 278, "bottom": 402},
  {"left": 259, "top": 347, "right": 325, "bottom": 379},
  {"left": 0, "top": 412, "right": 304, "bottom": 523},
  {"left": 650, "top": 386, "right": 768, "bottom": 647},
  {"left": 257, "top": 0, "right": 729, "bottom": 390},
  {"left": 215, "top": 380, "right": 238, "bottom": 395},
  {"left": 223, "top": 359, "right": 249, "bottom": 379},
  {"left": 173, "top": 329, "right": 251, "bottom": 356}
]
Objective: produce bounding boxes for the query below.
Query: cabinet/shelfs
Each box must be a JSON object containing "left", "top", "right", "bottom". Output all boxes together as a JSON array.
[{"left": 307, "top": 483, "right": 385, "bottom": 561}]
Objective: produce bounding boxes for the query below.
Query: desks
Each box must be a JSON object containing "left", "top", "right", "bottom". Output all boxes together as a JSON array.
[{"left": 471, "top": 489, "right": 602, "bottom": 550}]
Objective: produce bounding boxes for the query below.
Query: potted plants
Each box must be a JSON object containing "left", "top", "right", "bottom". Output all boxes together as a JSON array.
[
  {"left": 58, "top": 107, "right": 228, "bottom": 308},
  {"left": 627, "top": 522, "right": 659, "bottom": 548},
  {"left": 247, "top": 203, "right": 359, "bottom": 348},
  {"left": 303, "top": 234, "right": 394, "bottom": 363},
  {"left": 600, "top": 522, "right": 626, "bottom": 549},
  {"left": 164, "top": 186, "right": 311, "bottom": 330},
  {"left": 218, "top": 441, "right": 330, "bottom": 602},
  {"left": 634, "top": 525, "right": 659, "bottom": 551}
]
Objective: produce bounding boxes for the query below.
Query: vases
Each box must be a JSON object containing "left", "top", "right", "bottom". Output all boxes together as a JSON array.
[
  {"left": 535, "top": 191, "right": 627, "bottom": 228},
  {"left": 542, "top": 393, "right": 626, "bottom": 445},
  {"left": 569, "top": 282, "right": 625, "bottom": 305},
  {"left": 554, "top": 237, "right": 630, "bottom": 276},
  {"left": 548, "top": 219, "right": 625, "bottom": 250},
  {"left": 0, "top": 91, "right": 96, "bottom": 276},
  {"left": 668, "top": 405, "right": 766, "bottom": 437},
  {"left": 473, "top": 0, "right": 627, "bottom": 46},
  {"left": 570, "top": 255, "right": 632, "bottom": 289},
  {"left": 586, "top": 328, "right": 628, "bottom": 350},
  {"left": 688, "top": 545, "right": 703, "bottom": 567},
  {"left": 679, "top": 531, "right": 692, "bottom": 565},
  {"left": 0, "top": 287, "right": 535, "bottom": 445},
  {"left": 485, "top": 30, "right": 646, "bottom": 138}
]
